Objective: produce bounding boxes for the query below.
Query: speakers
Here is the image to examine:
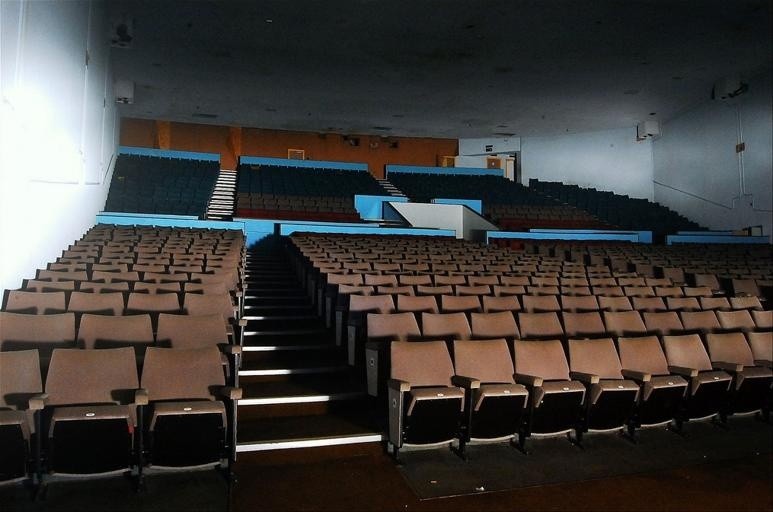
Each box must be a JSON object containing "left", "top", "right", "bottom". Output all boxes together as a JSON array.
[
  {"left": 114, "top": 80, "right": 135, "bottom": 105},
  {"left": 714, "top": 75, "right": 743, "bottom": 99},
  {"left": 637, "top": 121, "right": 660, "bottom": 139}
]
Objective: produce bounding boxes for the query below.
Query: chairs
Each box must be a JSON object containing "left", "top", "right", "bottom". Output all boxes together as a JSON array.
[
  {"left": 288, "top": 230, "right": 773, "bottom": 469},
  {"left": 0, "top": 222, "right": 249, "bottom": 511},
  {"left": 105, "top": 152, "right": 714, "bottom": 232}
]
